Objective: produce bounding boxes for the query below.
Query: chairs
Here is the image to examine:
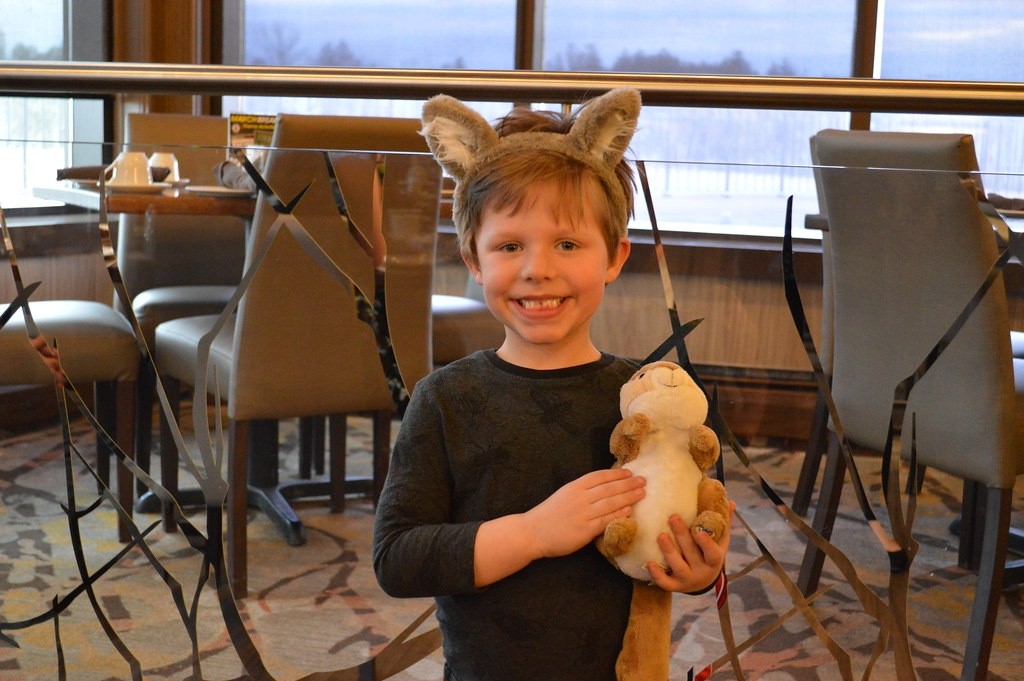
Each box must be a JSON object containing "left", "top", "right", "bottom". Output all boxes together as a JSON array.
[
  {"left": 790, "top": 127, "right": 1024, "bottom": 681},
  {"left": 0, "top": 298, "right": 143, "bottom": 544},
  {"left": 791, "top": 136, "right": 925, "bottom": 518},
  {"left": 109, "top": 112, "right": 250, "bottom": 512},
  {"left": 430, "top": 264, "right": 508, "bottom": 368},
  {"left": 156, "top": 113, "right": 445, "bottom": 601}
]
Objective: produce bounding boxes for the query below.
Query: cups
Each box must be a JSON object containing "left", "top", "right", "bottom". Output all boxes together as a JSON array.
[
  {"left": 100, "top": 152, "right": 152, "bottom": 185},
  {"left": 150, "top": 166, "right": 172, "bottom": 181},
  {"left": 149, "top": 152, "right": 180, "bottom": 181}
]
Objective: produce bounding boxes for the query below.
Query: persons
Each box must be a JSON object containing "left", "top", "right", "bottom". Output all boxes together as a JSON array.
[{"left": 370, "top": 105, "right": 736, "bottom": 681}]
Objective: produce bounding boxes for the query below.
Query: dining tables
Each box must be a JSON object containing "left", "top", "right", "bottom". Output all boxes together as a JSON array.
[{"left": 36, "top": 178, "right": 256, "bottom": 216}]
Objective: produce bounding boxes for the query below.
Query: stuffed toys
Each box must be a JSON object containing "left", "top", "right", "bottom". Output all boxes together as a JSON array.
[{"left": 595, "top": 361, "right": 729, "bottom": 681}]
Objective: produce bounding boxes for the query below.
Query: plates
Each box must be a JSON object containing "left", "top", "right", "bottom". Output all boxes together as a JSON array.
[
  {"left": 94, "top": 181, "right": 173, "bottom": 192},
  {"left": 186, "top": 183, "right": 256, "bottom": 200},
  {"left": 162, "top": 179, "right": 192, "bottom": 186}
]
